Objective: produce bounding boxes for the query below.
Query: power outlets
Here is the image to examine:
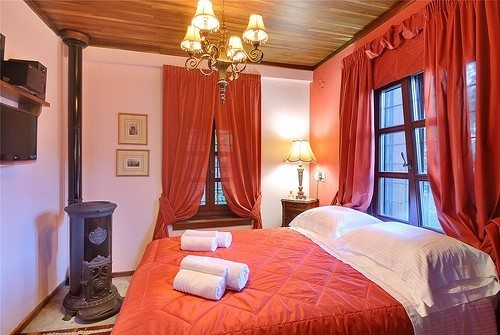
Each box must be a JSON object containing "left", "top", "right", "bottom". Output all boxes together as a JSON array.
[{"left": 315, "top": 172, "right": 326, "bottom": 182}]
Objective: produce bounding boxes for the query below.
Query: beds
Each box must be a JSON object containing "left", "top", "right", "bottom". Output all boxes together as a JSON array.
[{"left": 108, "top": 227, "right": 500, "bottom": 335}]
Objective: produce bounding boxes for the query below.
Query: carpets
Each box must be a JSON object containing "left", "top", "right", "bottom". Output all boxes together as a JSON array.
[{"left": 20, "top": 276, "right": 133, "bottom": 334}]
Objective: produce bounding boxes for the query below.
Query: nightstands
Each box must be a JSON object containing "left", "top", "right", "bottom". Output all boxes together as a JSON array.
[{"left": 281, "top": 199, "right": 319, "bottom": 227}]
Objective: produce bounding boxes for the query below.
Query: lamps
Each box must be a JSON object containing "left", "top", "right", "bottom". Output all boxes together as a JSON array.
[
  {"left": 180, "top": 0, "right": 268, "bottom": 104},
  {"left": 283, "top": 140, "right": 318, "bottom": 199}
]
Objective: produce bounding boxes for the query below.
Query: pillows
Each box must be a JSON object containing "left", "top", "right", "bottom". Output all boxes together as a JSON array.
[
  {"left": 288, "top": 206, "right": 383, "bottom": 241},
  {"left": 331, "top": 221, "right": 500, "bottom": 307}
]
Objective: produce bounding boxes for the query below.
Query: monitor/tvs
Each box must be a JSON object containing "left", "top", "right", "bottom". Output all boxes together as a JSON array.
[{"left": 0, "top": 103, "right": 38, "bottom": 160}]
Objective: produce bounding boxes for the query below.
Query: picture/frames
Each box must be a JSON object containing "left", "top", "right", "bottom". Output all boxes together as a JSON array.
[
  {"left": 118, "top": 113, "right": 148, "bottom": 145},
  {"left": 115, "top": 149, "right": 150, "bottom": 177}
]
